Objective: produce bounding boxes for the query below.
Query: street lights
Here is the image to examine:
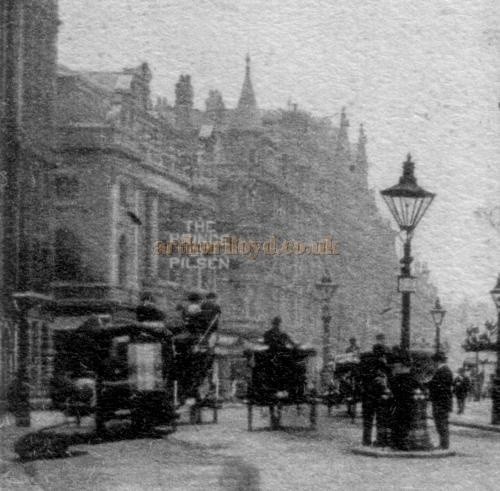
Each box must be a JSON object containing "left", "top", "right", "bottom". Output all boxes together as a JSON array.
[
  {"left": 430, "top": 298, "right": 448, "bottom": 351},
  {"left": 378, "top": 151, "right": 439, "bottom": 350}
]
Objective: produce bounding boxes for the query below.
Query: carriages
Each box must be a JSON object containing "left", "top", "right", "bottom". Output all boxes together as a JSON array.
[
  {"left": 233, "top": 336, "right": 323, "bottom": 431},
  {"left": 327, "top": 352, "right": 365, "bottom": 425}
]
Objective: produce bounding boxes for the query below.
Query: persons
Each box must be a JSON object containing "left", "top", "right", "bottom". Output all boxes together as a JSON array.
[
  {"left": 346, "top": 338, "right": 485, "bottom": 451},
  {"left": 135, "top": 291, "right": 220, "bottom": 333},
  {"left": 262, "top": 316, "right": 298, "bottom": 397}
]
